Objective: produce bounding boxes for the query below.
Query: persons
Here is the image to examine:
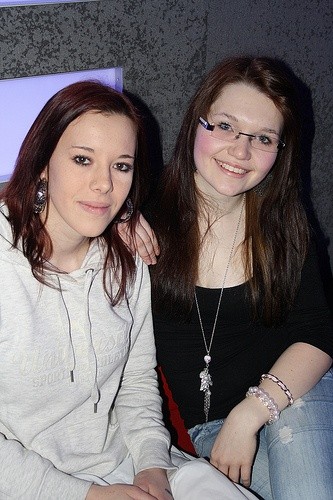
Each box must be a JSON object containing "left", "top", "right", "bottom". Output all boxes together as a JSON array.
[
  {"left": 0, "top": 77, "right": 261, "bottom": 500},
  {"left": 108, "top": 51, "right": 333, "bottom": 500}
]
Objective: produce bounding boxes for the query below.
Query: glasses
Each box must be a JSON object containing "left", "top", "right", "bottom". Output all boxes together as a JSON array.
[{"left": 199, "top": 118, "right": 285, "bottom": 153}]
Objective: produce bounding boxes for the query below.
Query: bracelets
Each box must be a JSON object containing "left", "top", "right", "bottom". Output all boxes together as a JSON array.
[
  {"left": 259, "top": 373, "right": 296, "bottom": 405},
  {"left": 244, "top": 386, "right": 282, "bottom": 425}
]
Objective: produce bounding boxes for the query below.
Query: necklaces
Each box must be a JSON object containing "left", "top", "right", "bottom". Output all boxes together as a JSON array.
[{"left": 192, "top": 194, "right": 246, "bottom": 422}]
